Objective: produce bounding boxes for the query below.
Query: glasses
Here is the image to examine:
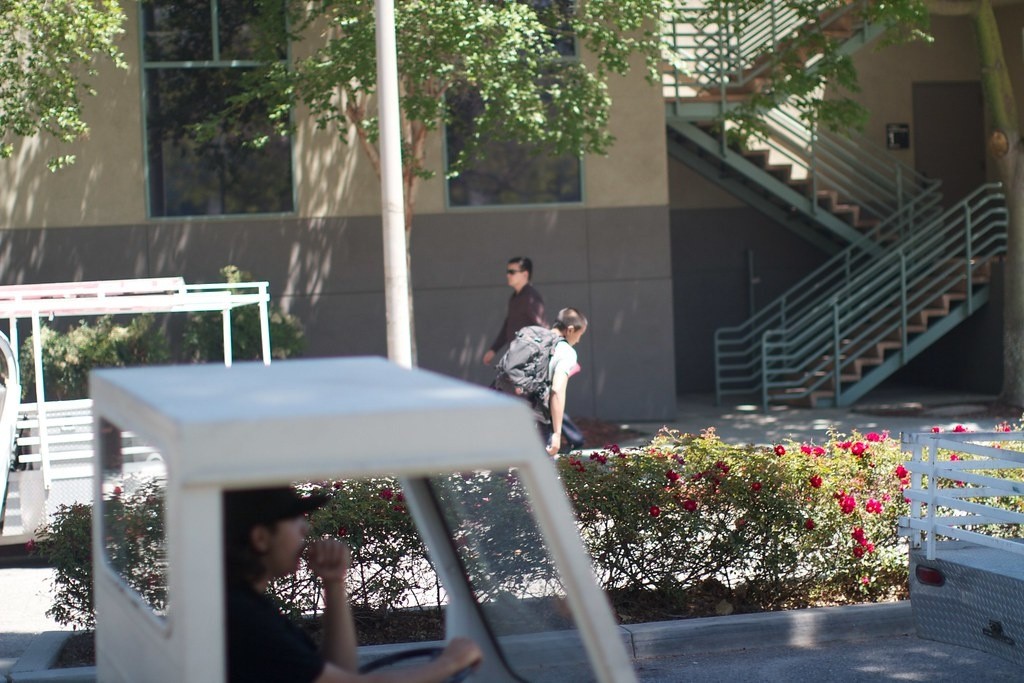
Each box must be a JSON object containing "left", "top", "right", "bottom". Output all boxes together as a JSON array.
[{"left": 505, "top": 269, "right": 522, "bottom": 275}]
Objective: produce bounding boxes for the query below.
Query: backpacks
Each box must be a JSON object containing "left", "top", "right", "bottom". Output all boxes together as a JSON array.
[{"left": 494, "top": 325, "right": 567, "bottom": 402}]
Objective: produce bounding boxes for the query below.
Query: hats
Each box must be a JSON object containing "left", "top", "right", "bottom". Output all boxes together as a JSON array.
[{"left": 222, "top": 487, "right": 331, "bottom": 538}]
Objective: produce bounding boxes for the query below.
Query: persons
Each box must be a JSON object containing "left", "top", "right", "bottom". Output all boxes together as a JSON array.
[
  {"left": 221, "top": 487, "right": 482, "bottom": 683},
  {"left": 532, "top": 308, "right": 589, "bottom": 456},
  {"left": 480, "top": 257, "right": 585, "bottom": 449}
]
